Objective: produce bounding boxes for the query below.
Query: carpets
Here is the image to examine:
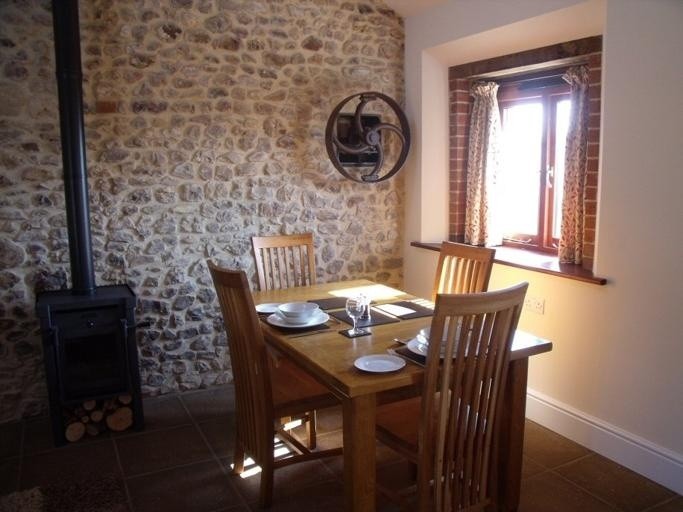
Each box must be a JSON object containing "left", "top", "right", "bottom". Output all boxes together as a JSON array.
[{"left": 1, "top": 468, "right": 128, "bottom": 512}]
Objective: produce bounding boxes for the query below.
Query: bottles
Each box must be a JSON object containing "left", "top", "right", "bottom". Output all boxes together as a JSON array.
[{"left": 360, "top": 294, "right": 372, "bottom": 320}]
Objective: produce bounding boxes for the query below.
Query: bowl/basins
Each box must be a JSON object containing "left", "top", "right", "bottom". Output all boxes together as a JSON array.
[
  {"left": 418, "top": 325, "right": 461, "bottom": 353},
  {"left": 278, "top": 302, "right": 320, "bottom": 324}
]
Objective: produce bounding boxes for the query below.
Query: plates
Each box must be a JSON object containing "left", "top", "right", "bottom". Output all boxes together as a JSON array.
[
  {"left": 407, "top": 332, "right": 488, "bottom": 359},
  {"left": 266, "top": 312, "right": 331, "bottom": 328},
  {"left": 352, "top": 354, "right": 407, "bottom": 373},
  {"left": 255, "top": 303, "right": 282, "bottom": 314}
]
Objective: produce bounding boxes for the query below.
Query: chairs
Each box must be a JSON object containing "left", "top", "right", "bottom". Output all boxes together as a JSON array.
[
  {"left": 206, "top": 260, "right": 343, "bottom": 508},
  {"left": 432, "top": 240, "right": 496, "bottom": 303},
  {"left": 375, "top": 281, "right": 529, "bottom": 512},
  {"left": 251, "top": 232, "right": 317, "bottom": 291}
]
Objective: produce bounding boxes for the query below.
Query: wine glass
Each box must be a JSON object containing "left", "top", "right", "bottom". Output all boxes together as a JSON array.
[{"left": 345, "top": 297, "right": 366, "bottom": 336}]
